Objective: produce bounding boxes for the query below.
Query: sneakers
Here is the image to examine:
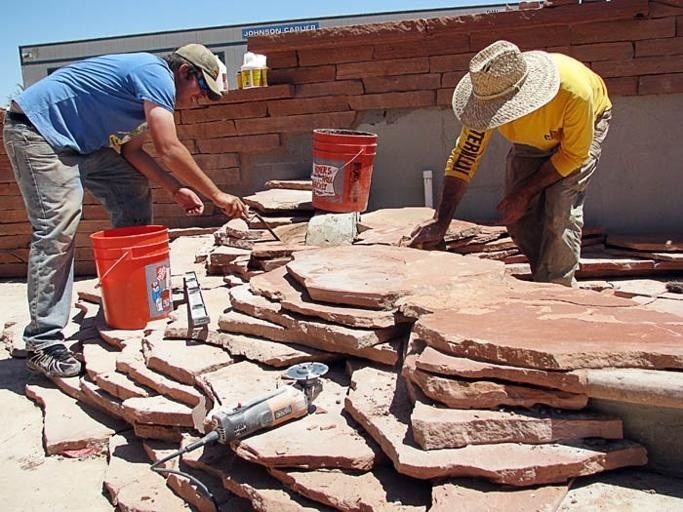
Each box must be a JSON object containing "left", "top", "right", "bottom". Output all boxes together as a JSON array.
[{"left": 25, "top": 344, "right": 81, "bottom": 377}]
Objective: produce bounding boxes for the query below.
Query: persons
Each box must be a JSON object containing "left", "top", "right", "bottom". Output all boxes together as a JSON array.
[
  {"left": 2, "top": 42, "right": 252, "bottom": 378},
  {"left": 407, "top": 39, "right": 612, "bottom": 289}
]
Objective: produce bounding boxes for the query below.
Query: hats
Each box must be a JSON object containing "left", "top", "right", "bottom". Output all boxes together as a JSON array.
[
  {"left": 452, "top": 38, "right": 559, "bottom": 133},
  {"left": 175, "top": 44, "right": 223, "bottom": 101}
]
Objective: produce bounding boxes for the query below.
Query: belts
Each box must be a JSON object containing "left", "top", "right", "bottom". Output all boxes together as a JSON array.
[{"left": 3, "top": 112, "right": 31, "bottom": 125}]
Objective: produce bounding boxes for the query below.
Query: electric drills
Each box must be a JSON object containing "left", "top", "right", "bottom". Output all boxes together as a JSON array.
[{"left": 148, "top": 362, "right": 329, "bottom": 469}]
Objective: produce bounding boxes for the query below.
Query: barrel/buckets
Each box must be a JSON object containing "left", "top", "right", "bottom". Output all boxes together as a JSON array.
[
  {"left": 311, "top": 129, "right": 378, "bottom": 212},
  {"left": 93, "top": 224, "right": 171, "bottom": 328}
]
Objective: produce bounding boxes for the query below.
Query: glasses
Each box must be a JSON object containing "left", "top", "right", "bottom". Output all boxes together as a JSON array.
[{"left": 192, "top": 69, "right": 209, "bottom": 92}]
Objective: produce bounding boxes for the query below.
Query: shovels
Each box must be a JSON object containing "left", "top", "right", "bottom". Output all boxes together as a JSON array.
[{"left": 241, "top": 211, "right": 281, "bottom": 241}]
